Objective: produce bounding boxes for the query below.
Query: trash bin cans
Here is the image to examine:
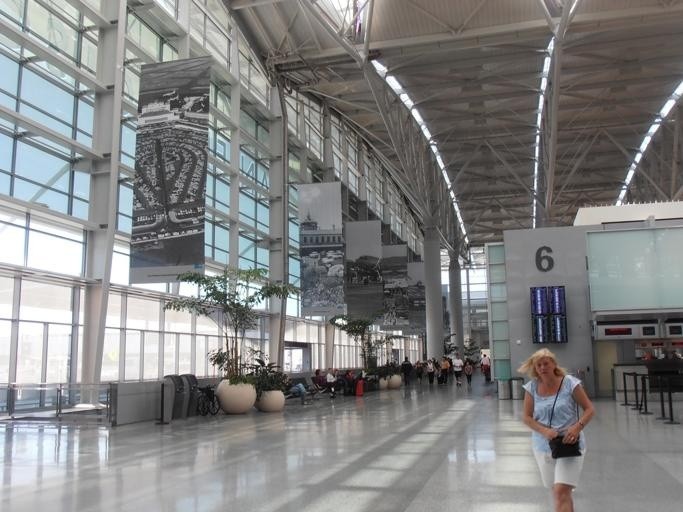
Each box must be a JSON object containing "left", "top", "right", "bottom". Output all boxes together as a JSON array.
[
  {"left": 498, "top": 379, "right": 510, "bottom": 400},
  {"left": 511, "top": 377, "right": 524, "bottom": 400}
]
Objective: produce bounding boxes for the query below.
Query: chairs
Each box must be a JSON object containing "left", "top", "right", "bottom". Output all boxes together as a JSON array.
[{"left": 280, "top": 375, "right": 351, "bottom": 400}]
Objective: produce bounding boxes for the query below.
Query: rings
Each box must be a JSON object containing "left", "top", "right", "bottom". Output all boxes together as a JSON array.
[{"left": 571, "top": 437, "right": 574, "bottom": 441}]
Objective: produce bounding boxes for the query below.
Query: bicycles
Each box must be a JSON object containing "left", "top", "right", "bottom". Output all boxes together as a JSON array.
[{"left": 196, "top": 384, "right": 220, "bottom": 417}]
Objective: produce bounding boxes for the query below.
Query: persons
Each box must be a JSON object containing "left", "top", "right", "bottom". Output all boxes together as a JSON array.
[
  {"left": 517, "top": 348, "right": 596, "bottom": 512},
  {"left": 313, "top": 368, "right": 354, "bottom": 399},
  {"left": 400, "top": 353, "right": 490, "bottom": 387},
  {"left": 281, "top": 382, "right": 310, "bottom": 405}
]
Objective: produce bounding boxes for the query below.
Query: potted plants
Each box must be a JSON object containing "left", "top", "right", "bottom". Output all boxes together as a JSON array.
[
  {"left": 334, "top": 309, "right": 402, "bottom": 390},
  {"left": 163, "top": 266, "right": 302, "bottom": 415},
  {"left": 250, "top": 358, "right": 289, "bottom": 412}
]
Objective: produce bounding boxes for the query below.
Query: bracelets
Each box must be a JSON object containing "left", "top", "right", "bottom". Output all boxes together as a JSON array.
[{"left": 578, "top": 421, "right": 584, "bottom": 428}]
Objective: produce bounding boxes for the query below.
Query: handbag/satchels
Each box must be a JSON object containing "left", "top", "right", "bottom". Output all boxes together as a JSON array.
[{"left": 548, "top": 436, "right": 582, "bottom": 458}]
